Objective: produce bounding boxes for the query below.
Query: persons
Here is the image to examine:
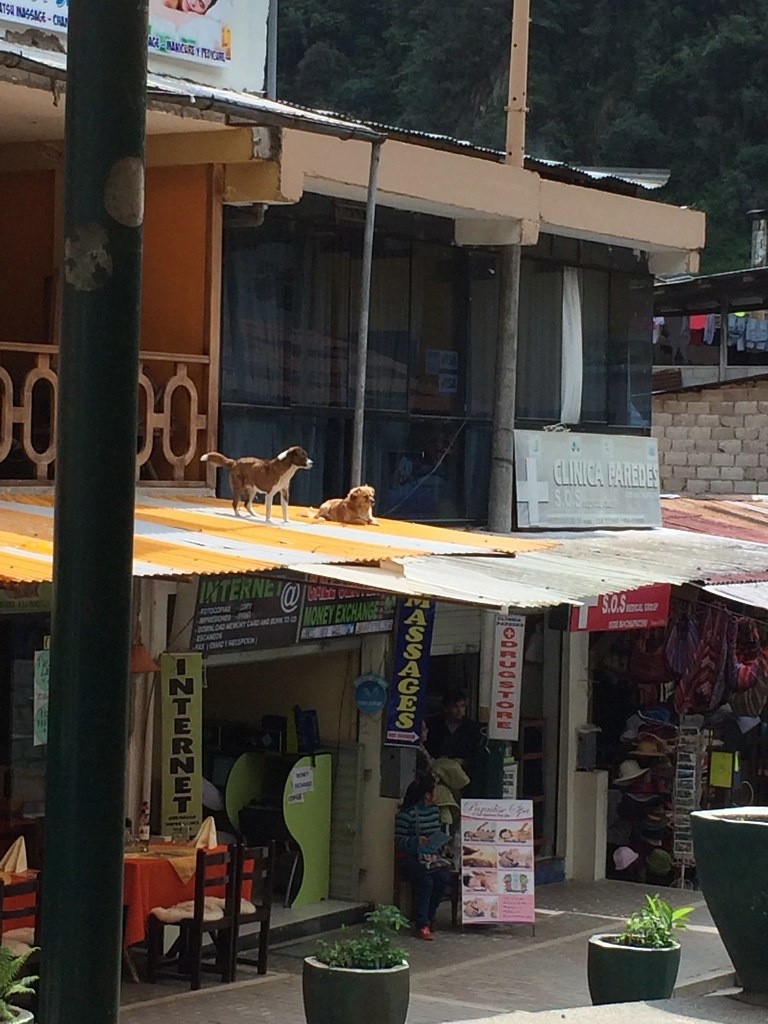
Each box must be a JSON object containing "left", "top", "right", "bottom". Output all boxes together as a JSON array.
[
  {"left": 395, "top": 781, "right": 450, "bottom": 941},
  {"left": 422, "top": 689, "right": 480, "bottom": 761}
]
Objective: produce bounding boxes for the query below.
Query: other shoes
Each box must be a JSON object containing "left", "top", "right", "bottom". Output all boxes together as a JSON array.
[{"left": 415, "top": 920, "right": 434, "bottom": 940}]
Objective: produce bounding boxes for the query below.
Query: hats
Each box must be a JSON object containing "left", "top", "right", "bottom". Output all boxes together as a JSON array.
[
  {"left": 613, "top": 846, "right": 639, "bottom": 870},
  {"left": 620, "top": 714, "right": 646, "bottom": 742},
  {"left": 609, "top": 816, "right": 633, "bottom": 841},
  {"left": 629, "top": 738, "right": 666, "bottom": 758},
  {"left": 638, "top": 724, "right": 675, "bottom": 742},
  {"left": 736, "top": 716, "right": 761, "bottom": 735},
  {"left": 646, "top": 849, "right": 672, "bottom": 872},
  {"left": 638, "top": 775, "right": 672, "bottom": 794},
  {"left": 639, "top": 817, "right": 671, "bottom": 841},
  {"left": 613, "top": 760, "right": 650, "bottom": 786},
  {"left": 637, "top": 706, "right": 673, "bottom": 727}
]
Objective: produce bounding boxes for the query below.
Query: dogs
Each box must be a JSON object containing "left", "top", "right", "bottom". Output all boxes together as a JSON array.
[
  {"left": 199, "top": 445, "right": 314, "bottom": 525},
  {"left": 314, "top": 484, "right": 382, "bottom": 528}
]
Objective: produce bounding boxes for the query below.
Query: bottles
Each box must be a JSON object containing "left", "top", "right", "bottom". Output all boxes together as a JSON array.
[{"left": 138, "top": 814, "right": 150, "bottom": 853}]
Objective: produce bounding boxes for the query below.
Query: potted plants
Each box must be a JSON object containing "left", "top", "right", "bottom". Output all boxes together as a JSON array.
[
  {"left": 588, "top": 891, "right": 693, "bottom": 1005},
  {"left": 302, "top": 905, "right": 410, "bottom": 1024},
  {"left": 0, "top": 944, "right": 41, "bottom": 1024}
]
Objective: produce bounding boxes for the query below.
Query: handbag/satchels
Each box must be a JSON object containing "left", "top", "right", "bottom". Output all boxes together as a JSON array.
[
  {"left": 418, "top": 851, "right": 451, "bottom": 874},
  {"left": 605, "top": 597, "right": 768, "bottom": 717}
]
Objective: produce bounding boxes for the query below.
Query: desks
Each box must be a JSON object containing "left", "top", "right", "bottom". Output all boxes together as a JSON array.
[{"left": 122, "top": 837, "right": 254, "bottom": 983}]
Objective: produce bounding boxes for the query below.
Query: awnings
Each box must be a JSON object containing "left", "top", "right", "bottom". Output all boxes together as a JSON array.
[{"left": 0, "top": 496, "right": 768, "bottom": 608}]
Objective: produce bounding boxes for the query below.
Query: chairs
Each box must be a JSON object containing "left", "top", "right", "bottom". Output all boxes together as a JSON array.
[
  {"left": 0, "top": 873, "right": 44, "bottom": 1023},
  {"left": 147, "top": 839, "right": 277, "bottom": 990}
]
